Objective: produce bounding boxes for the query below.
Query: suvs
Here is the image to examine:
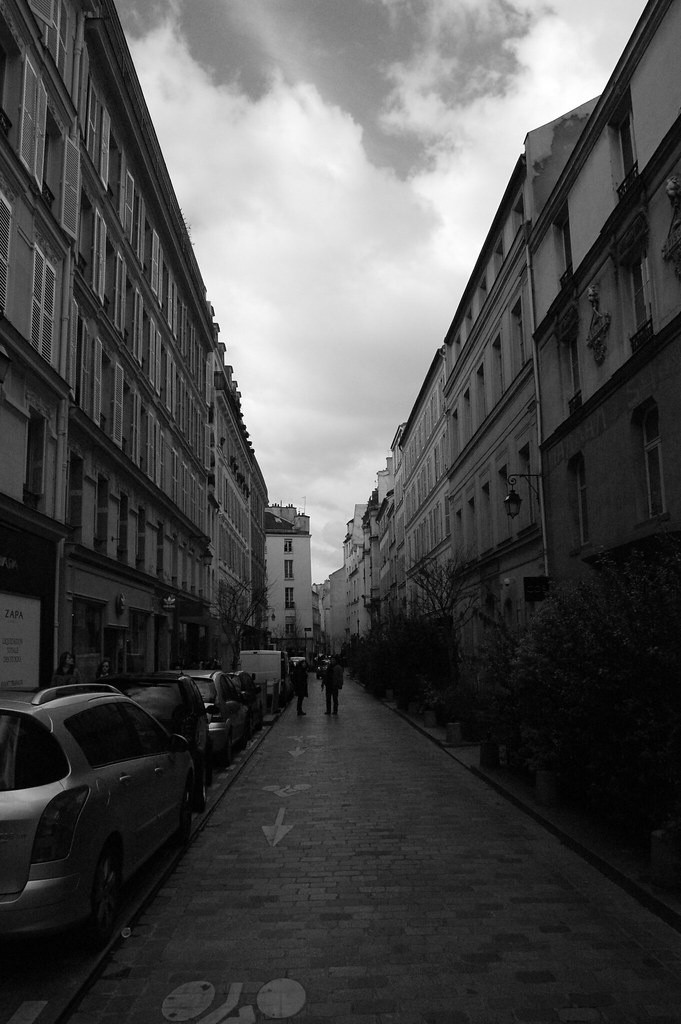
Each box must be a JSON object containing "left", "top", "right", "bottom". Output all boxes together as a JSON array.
[{"left": 0, "top": 683, "right": 196, "bottom": 947}]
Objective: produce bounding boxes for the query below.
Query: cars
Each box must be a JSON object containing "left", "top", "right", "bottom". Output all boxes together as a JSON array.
[
  {"left": 94, "top": 670, "right": 268, "bottom": 813},
  {"left": 316, "top": 658, "right": 329, "bottom": 680}
]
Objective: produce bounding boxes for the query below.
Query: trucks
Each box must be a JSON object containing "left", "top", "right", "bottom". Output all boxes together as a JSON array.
[{"left": 236, "top": 650, "right": 295, "bottom": 707}]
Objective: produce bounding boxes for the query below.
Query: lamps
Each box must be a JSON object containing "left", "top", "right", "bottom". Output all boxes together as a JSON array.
[
  {"left": 504, "top": 473, "right": 540, "bottom": 519},
  {"left": 188, "top": 537, "right": 213, "bottom": 566}
]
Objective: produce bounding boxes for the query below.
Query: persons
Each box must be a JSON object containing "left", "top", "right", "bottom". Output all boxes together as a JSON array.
[
  {"left": 98, "top": 661, "right": 111, "bottom": 678},
  {"left": 296, "top": 660, "right": 308, "bottom": 715},
  {"left": 50, "top": 652, "right": 81, "bottom": 686},
  {"left": 318, "top": 653, "right": 344, "bottom": 715}
]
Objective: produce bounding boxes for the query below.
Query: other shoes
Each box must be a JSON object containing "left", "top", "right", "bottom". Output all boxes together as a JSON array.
[
  {"left": 332, "top": 711, "right": 337, "bottom": 715},
  {"left": 297, "top": 712, "right": 306, "bottom": 715},
  {"left": 324, "top": 711, "right": 331, "bottom": 715}
]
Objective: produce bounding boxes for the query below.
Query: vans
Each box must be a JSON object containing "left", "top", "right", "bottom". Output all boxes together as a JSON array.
[{"left": 290, "top": 657, "right": 306, "bottom": 675}]
